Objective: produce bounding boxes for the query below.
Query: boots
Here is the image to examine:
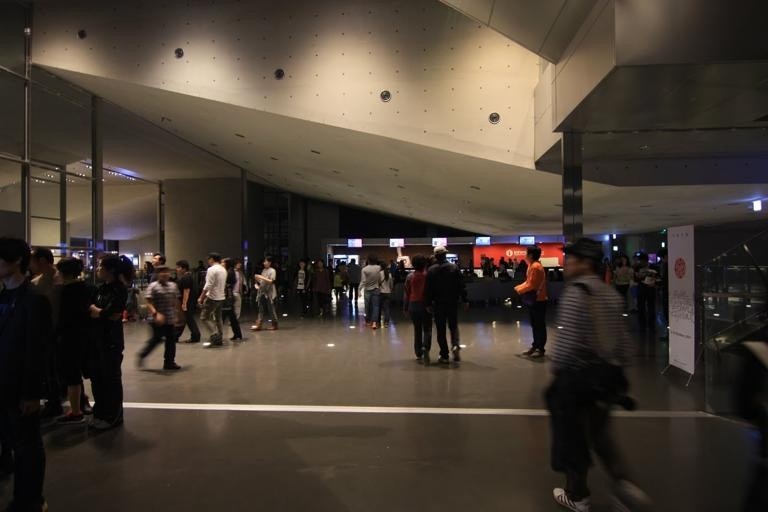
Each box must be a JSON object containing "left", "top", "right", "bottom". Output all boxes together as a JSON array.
[{"left": 250, "top": 320, "right": 279, "bottom": 330}]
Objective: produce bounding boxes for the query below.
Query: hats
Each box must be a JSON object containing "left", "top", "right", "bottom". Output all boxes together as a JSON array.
[
  {"left": 434, "top": 246, "right": 448, "bottom": 254},
  {"left": 561, "top": 238, "right": 603, "bottom": 260}
]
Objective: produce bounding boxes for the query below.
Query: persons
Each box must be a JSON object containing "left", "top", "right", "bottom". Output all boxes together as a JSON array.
[
  {"left": 250, "top": 256, "right": 279, "bottom": 330},
  {"left": 540, "top": 237, "right": 655, "bottom": 512},
  {"left": 468, "top": 253, "right": 564, "bottom": 307},
  {"left": 403, "top": 254, "right": 433, "bottom": 366},
  {"left": 294, "top": 254, "right": 409, "bottom": 329},
  {"left": 1, "top": 238, "right": 140, "bottom": 511},
  {"left": 514, "top": 245, "right": 548, "bottom": 357},
  {"left": 601, "top": 247, "right": 668, "bottom": 336},
  {"left": 424, "top": 245, "right": 469, "bottom": 363},
  {"left": 140, "top": 252, "right": 248, "bottom": 370}
]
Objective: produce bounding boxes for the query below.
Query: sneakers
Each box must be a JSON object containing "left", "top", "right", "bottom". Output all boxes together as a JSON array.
[
  {"left": 365, "top": 319, "right": 389, "bottom": 329},
  {"left": 208, "top": 333, "right": 241, "bottom": 346},
  {"left": 553, "top": 487, "right": 590, "bottom": 512},
  {"left": 417, "top": 346, "right": 459, "bottom": 364},
  {"left": 185, "top": 336, "right": 199, "bottom": 343},
  {"left": 41, "top": 405, "right": 122, "bottom": 430},
  {"left": 163, "top": 361, "right": 180, "bottom": 370},
  {"left": 523, "top": 349, "right": 542, "bottom": 358},
  {"left": 134, "top": 352, "right": 141, "bottom": 368}
]
